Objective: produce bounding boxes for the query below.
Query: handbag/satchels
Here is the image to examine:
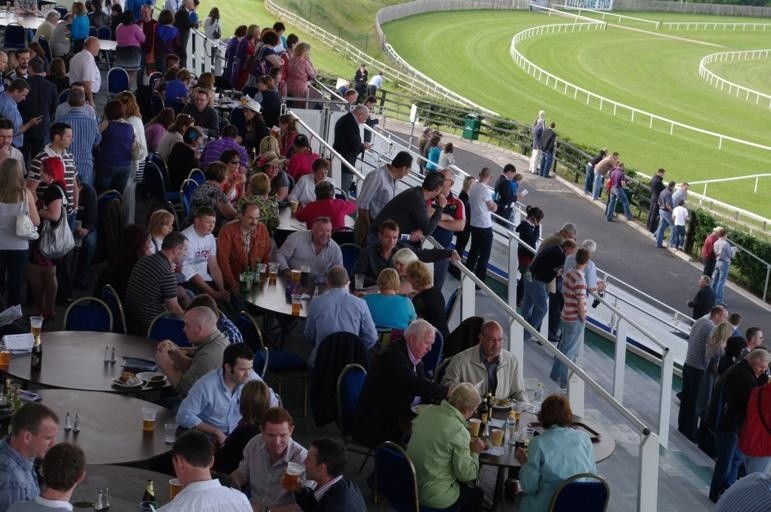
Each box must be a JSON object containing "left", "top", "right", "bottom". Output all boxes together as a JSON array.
[
  {"left": 16, "top": 214, "right": 40, "bottom": 240},
  {"left": 40, "top": 207, "right": 75, "bottom": 259}
]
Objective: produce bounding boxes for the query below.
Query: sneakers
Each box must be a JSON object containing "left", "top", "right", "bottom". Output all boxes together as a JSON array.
[{"left": 504, "top": 478, "right": 521, "bottom": 497}]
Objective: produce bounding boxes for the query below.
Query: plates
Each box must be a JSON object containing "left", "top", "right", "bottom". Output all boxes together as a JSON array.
[
  {"left": 409, "top": 404, "right": 437, "bottom": 414},
  {"left": 136, "top": 372, "right": 168, "bottom": 383},
  {"left": 112, "top": 376, "right": 144, "bottom": 388}
]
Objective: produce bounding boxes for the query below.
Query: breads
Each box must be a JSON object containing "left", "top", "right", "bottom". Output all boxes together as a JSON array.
[
  {"left": 120, "top": 371, "right": 138, "bottom": 386},
  {"left": 492, "top": 398, "right": 511, "bottom": 408},
  {"left": 162, "top": 341, "right": 170, "bottom": 351}
]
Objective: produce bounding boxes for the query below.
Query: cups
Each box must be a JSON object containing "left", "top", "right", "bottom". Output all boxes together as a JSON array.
[
  {"left": 139, "top": 501, "right": 159, "bottom": 512},
  {"left": 463, "top": 392, "right": 546, "bottom": 457},
  {"left": 164, "top": 424, "right": 178, "bottom": 443},
  {"left": 169, "top": 478, "right": 184, "bottom": 502},
  {"left": 0, "top": 345, "right": 11, "bottom": 366},
  {"left": 141, "top": 407, "right": 158, "bottom": 432},
  {"left": 237, "top": 258, "right": 367, "bottom": 317},
  {"left": 30, "top": 315, "right": 45, "bottom": 338},
  {"left": 17, "top": 17, "right": 24, "bottom": 23},
  {"left": 280, "top": 460, "right": 306, "bottom": 490},
  {"left": 289, "top": 201, "right": 299, "bottom": 213}
]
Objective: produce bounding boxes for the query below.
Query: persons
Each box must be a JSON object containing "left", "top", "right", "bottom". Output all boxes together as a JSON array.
[
  {"left": 583, "top": 148, "right": 633, "bottom": 223},
  {"left": 1, "top": 1, "right": 606, "bottom": 510},
  {"left": 679, "top": 275, "right": 770, "bottom": 511},
  {"left": 704, "top": 226, "right": 732, "bottom": 307},
  {"left": 647, "top": 169, "right": 691, "bottom": 250}
]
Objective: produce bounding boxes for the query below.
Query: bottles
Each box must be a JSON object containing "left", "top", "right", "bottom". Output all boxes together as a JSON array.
[
  {"left": 29, "top": 335, "right": 42, "bottom": 376},
  {"left": 98, "top": 488, "right": 105, "bottom": 512},
  {"left": 110, "top": 346, "right": 117, "bottom": 364},
  {"left": 104, "top": 346, "right": 110, "bottom": 362},
  {"left": 212, "top": 87, "right": 252, "bottom": 109},
  {"left": 64, "top": 412, "right": 73, "bottom": 432},
  {"left": 141, "top": 479, "right": 158, "bottom": 512},
  {"left": 94, "top": 492, "right": 104, "bottom": 512},
  {"left": 72, "top": 412, "right": 81, "bottom": 434},
  {"left": 532, "top": 382, "right": 543, "bottom": 418},
  {"left": 0, "top": 380, "right": 22, "bottom": 414},
  {"left": 248, "top": 146, "right": 256, "bottom": 166},
  {"left": 592, "top": 294, "right": 605, "bottom": 308},
  {"left": 102, "top": 487, "right": 111, "bottom": 512}
]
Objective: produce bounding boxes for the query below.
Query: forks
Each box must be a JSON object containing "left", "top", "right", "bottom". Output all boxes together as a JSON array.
[{"left": 144, "top": 376, "right": 152, "bottom": 387}]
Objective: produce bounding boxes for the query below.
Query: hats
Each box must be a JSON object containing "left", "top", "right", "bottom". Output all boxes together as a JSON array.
[{"left": 257, "top": 151, "right": 287, "bottom": 169}]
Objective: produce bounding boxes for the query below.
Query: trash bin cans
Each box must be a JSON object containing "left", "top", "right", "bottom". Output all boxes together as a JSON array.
[{"left": 463, "top": 112, "right": 481, "bottom": 140}]
